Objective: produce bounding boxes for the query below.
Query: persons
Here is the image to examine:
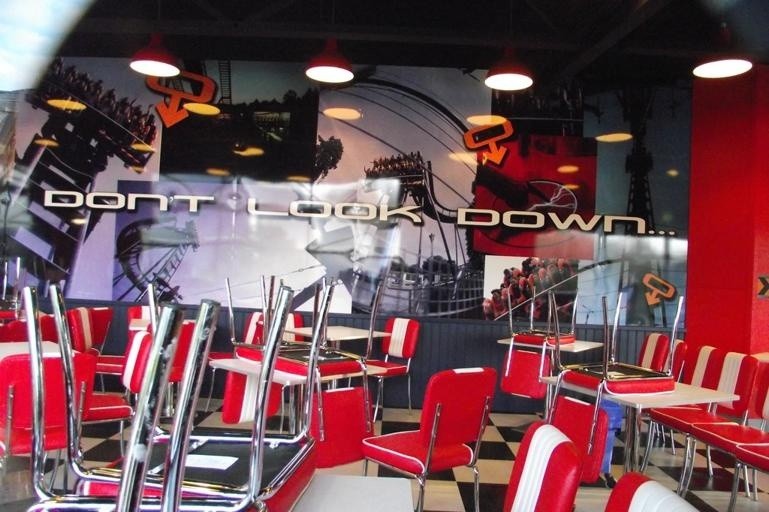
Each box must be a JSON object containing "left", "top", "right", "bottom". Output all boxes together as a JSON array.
[
  {"left": 35, "top": 54, "right": 160, "bottom": 165},
  {"left": 481, "top": 256, "right": 577, "bottom": 322},
  {"left": 361, "top": 150, "right": 429, "bottom": 190}
]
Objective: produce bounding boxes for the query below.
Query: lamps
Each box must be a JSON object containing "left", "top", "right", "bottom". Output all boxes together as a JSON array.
[
  {"left": 125, "top": 0, "right": 185, "bottom": 82},
  {"left": 691, "top": 1, "right": 757, "bottom": 80},
  {"left": 302, "top": 0, "right": 355, "bottom": 88},
  {"left": 482, "top": 0, "right": 536, "bottom": 94}
]
